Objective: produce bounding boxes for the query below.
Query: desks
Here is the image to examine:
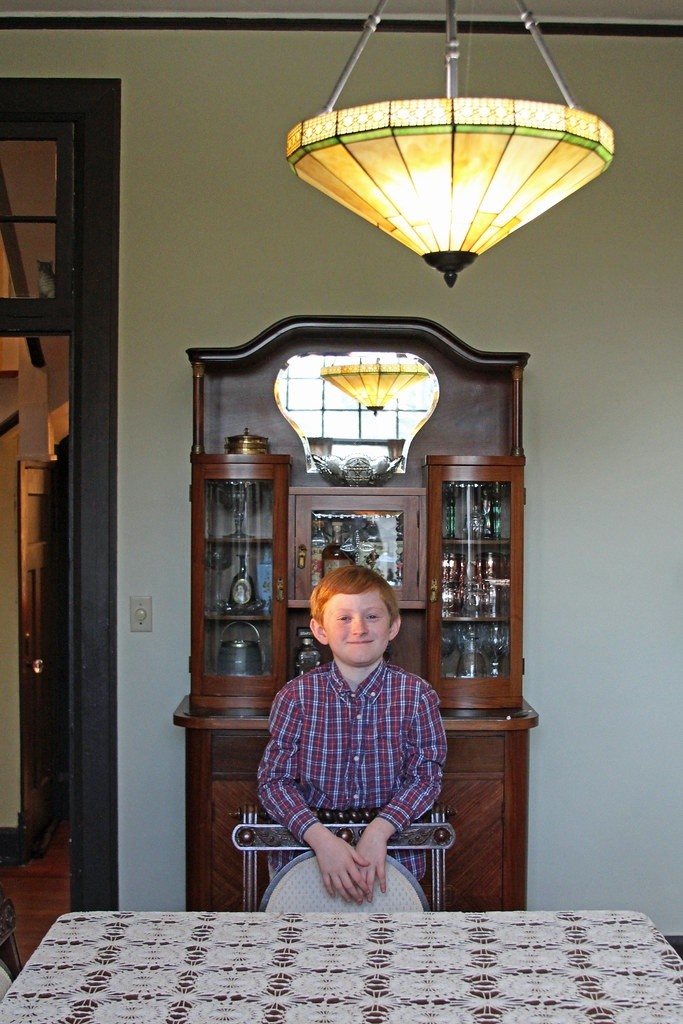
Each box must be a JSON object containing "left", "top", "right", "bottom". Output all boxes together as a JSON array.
[{"left": 0, "top": 910, "right": 683, "bottom": 1024}]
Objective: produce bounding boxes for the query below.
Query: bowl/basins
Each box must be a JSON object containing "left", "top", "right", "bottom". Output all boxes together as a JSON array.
[{"left": 312, "top": 453, "right": 405, "bottom": 487}]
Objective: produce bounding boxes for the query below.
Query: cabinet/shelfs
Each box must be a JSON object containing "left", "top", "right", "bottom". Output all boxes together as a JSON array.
[{"left": 170, "top": 315, "right": 540, "bottom": 914}]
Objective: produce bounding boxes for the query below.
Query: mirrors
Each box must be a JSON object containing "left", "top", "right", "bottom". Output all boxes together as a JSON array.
[{"left": 273, "top": 352, "right": 440, "bottom": 474}]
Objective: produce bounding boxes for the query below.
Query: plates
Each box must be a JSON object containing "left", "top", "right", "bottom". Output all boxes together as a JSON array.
[{"left": 215, "top": 599, "right": 266, "bottom": 615}]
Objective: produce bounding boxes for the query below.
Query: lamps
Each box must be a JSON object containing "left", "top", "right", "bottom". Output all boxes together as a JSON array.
[
  {"left": 285, "top": 0, "right": 613, "bottom": 288},
  {"left": 320, "top": 356, "right": 430, "bottom": 416}
]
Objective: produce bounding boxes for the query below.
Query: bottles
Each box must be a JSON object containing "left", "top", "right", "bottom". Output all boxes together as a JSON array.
[
  {"left": 311, "top": 520, "right": 329, "bottom": 590},
  {"left": 228, "top": 555, "right": 255, "bottom": 604},
  {"left": 389, "top": 521, "right": 404, "bottom": 587},
  {"left": 257, "top": 544, "right": 273, "bottom": 611},
  {"left": 354, "top": 520, "right": 388, "bottom": 581},
  {"left": 322, "top": 522, "right": 355, "bottom": 579},
  {"left": 296, "top": 639, "right": 323, "bottom": 678}
]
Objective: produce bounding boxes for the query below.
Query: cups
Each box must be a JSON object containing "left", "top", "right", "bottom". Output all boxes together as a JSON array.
[{"left": 442, "top": 552, "right": 507, "bottom": 618}]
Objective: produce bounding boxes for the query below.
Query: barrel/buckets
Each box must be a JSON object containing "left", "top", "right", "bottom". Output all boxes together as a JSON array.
[{"left": 217, "top": 621, "right": 267, "bottom": 675}]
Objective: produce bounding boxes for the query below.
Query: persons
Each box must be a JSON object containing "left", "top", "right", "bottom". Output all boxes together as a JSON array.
[{"left": 255, "top": 565, "right": 453, "bottom": 906}]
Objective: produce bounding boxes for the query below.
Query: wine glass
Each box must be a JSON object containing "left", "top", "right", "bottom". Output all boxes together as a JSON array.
[{"left": 440, "top": 622, "right": 509, "bottom": 678}]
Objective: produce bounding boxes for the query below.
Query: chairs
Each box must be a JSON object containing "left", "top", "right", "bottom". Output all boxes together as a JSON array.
[{"left": 227, "top": 802, "right": 458, "bottom": 912}]
